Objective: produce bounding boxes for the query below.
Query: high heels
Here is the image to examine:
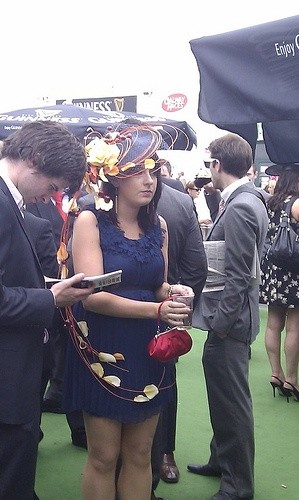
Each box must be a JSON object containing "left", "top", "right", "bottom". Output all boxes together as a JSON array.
[
  {"left": 270, "top": 376, "right": 292, "bottom": 398},
  {"left": 281, "top": 381, "right": 299, "bottom": 403}
]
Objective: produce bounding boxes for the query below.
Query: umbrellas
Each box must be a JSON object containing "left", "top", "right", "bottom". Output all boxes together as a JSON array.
[
  {"left": 189, "top": 14, "right": 299, "bottom": 164},
  {"left": 0, "top": 104, "right": 197, "bottom": 151}
]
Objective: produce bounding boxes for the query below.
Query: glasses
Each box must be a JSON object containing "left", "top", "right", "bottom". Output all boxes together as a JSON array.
[
  {"left": 192, "top": 188, "right": 201, "bottom": 192},
  {"left": 203, "top": 159, "right": 220, "bottom": 168}
]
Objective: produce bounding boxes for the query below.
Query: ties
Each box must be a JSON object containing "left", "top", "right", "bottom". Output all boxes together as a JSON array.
[{"left": 219, "top": 200, "right": 226, "bottom": 213}]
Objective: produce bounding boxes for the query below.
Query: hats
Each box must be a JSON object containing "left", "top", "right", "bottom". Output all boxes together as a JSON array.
[
  {"left": 85, "top": 123, "right": 189, "bottom": 178},
  {"left": 265, "top": 162, "right": 299, "bottom": 176}
]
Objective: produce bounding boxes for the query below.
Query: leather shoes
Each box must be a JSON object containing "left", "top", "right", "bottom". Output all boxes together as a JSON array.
[
  {"left": 187, "top": 464, "right": 218, "bottom": 476},
  {"left": 160, "top": 454, "right": 179, "bottom": 484},
  {"left": 210, "top": 491, "right": 254, "bottom": 500}
]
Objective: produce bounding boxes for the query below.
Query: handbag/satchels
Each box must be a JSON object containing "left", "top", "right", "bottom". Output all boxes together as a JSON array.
[
  {"left": 268, "top": 196, "right": 299, "bottom": 272},
  {"left": 148, "top": 297, "right": 193, "bottom": 358}
]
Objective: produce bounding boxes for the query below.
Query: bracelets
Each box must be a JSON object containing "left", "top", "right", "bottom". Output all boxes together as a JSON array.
[{"left": 167, "top": 285, "right": 174, "bottom": 297}]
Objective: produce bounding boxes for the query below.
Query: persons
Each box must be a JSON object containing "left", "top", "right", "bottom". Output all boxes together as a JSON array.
[
  {"left": 25, "top": 160, "right": 208, "bottom": 483},
  {"left": 188, "top": 136, "right": 269, "bottom": 500},
  {"left": 248, "top": 166, "right": 299, "bottom": 403},
  {"left": 73, "top": 123, "right": 194, "bottom": 500},
  {"left": 0, "top": 121, "right": 95, "bottom": 500}
]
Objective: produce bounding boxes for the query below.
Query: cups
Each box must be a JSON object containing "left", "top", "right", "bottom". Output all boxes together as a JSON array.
[
  {"left": 172, "top": 293, "right": 194, "bottom": 330},
  {"left": 193, "top": 169, "right": 212, "bottom": 189}
]
